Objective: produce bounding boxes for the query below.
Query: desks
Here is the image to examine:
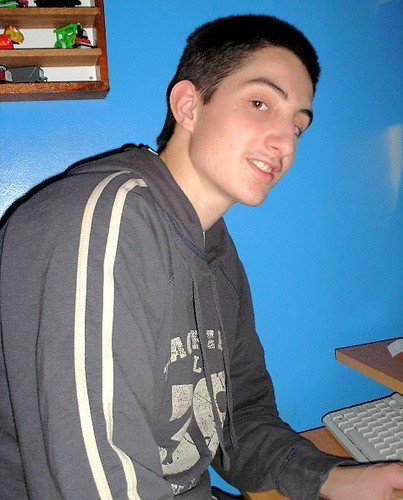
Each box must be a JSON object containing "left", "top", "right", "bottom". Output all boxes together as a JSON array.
[{"left": 237, "top": 338, "right": 403, "bottom": 500}]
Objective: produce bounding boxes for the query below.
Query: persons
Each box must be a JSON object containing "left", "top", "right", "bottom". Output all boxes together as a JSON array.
[{"left": 0, "top": 14, "right": 403, "bottom": 500}]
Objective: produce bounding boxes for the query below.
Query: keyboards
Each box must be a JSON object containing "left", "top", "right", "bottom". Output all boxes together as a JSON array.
[{"left": 322, "top": 392, "right": 403, "bottom": 467}]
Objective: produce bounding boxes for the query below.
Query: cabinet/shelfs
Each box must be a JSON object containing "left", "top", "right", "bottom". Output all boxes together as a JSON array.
[{"left": 0, "top": 0, "right": 110, "bottom": 102}]
